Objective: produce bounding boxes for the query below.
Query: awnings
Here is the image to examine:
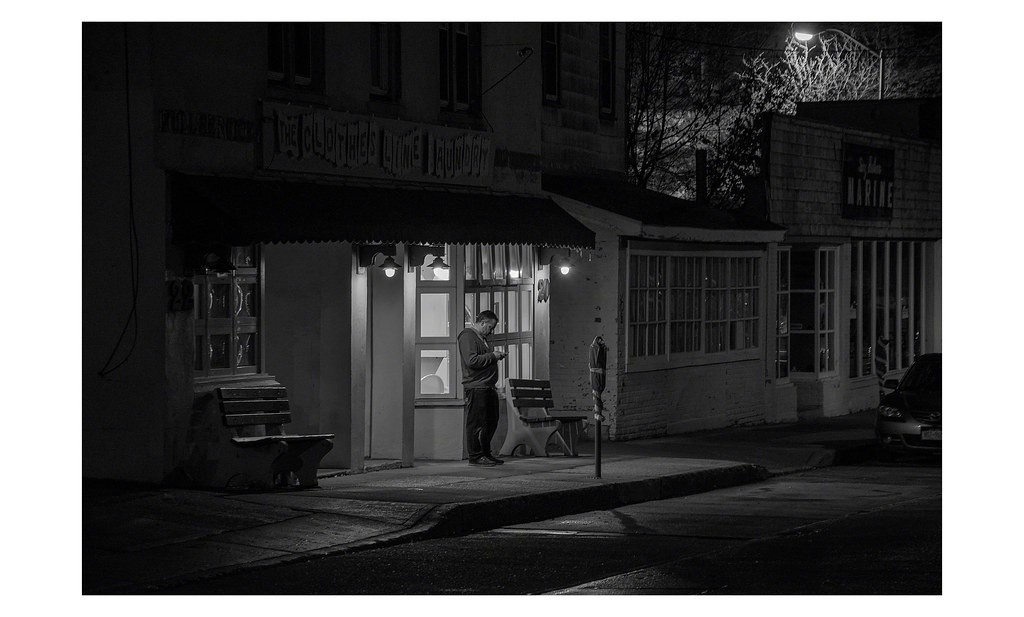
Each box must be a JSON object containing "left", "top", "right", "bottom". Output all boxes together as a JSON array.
[{"left": 216, "top": 175, "right": 598, "bottom": 251}]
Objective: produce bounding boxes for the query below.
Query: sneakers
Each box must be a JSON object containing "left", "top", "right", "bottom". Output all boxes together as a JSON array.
[
  {"left": 486, "top": 454, "right": 504, "bottom": 465},
  {"left": 469, "top": 456, "right": 496, "bottom": 467}
]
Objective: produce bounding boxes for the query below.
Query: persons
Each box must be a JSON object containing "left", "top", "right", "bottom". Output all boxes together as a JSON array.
[{"left": 456, "top": 310, "right": 509, "bottom": 467}]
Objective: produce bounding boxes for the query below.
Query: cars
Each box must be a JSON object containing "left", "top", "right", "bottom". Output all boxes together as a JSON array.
[{"left": 876, "top": 353, "right": 942, "bottom": 466}]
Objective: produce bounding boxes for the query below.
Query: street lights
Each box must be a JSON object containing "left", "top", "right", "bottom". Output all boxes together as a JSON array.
[{"left": 795, "top": 27, "right": 884, "bottom": 99}]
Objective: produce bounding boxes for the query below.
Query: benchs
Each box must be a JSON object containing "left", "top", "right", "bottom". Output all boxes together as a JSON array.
[
  {"left": 187, "top": 387, "right": 336, "bottom": 490},
  {"left": 499, "top": 378, "right": 588, "bottom": 457}
]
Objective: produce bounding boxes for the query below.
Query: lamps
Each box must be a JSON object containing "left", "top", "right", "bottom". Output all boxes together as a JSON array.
[
  {"left": 554, "top": 257, "right": 576, "bottom": 276},
  {"left": 205, "top": 248, "right": 239, "bottom": 275},
  {"left": 426, "top": 245, "right": 451, "bottom": 277},
  {"left": 481, "top": 47, "right": 534, "bottom": 96},
  {"left": 378, "top": 246, "right": 403, "bottom": 277}
]
